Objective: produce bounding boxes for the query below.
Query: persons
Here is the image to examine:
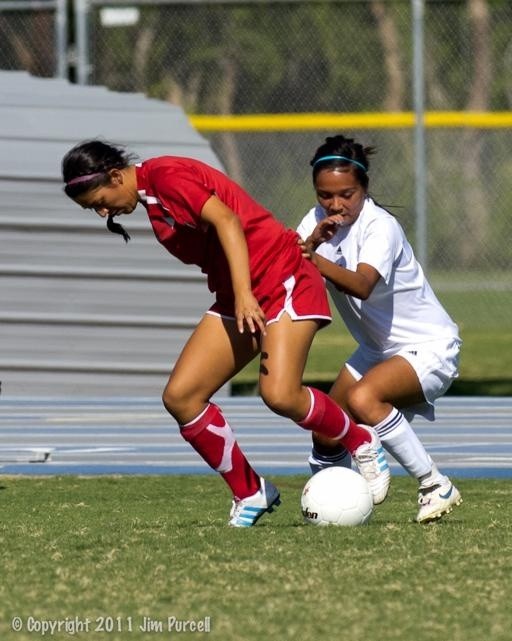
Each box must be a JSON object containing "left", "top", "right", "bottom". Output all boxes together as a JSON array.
[
  {"left": 295, "top": 134, "right": 463, "bottom": 524},
  {"left": 62, "top": 140, "right": 391, "bottom": 528}
]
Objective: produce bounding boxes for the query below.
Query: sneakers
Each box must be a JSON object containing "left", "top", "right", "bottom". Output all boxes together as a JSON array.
[
  {"left": 416, "top": 476, "right": 462, "bottom": 522},
  {"left": 353, "top": 424, "right": 390, "bottom": 504},
  {"left": 228, "top": 476, "right": 281, "bottom": 528}
]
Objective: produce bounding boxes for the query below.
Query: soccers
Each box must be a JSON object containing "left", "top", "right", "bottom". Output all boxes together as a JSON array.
[{"left": 300, "top": 467, "right": 373, "bottom": 526}]
